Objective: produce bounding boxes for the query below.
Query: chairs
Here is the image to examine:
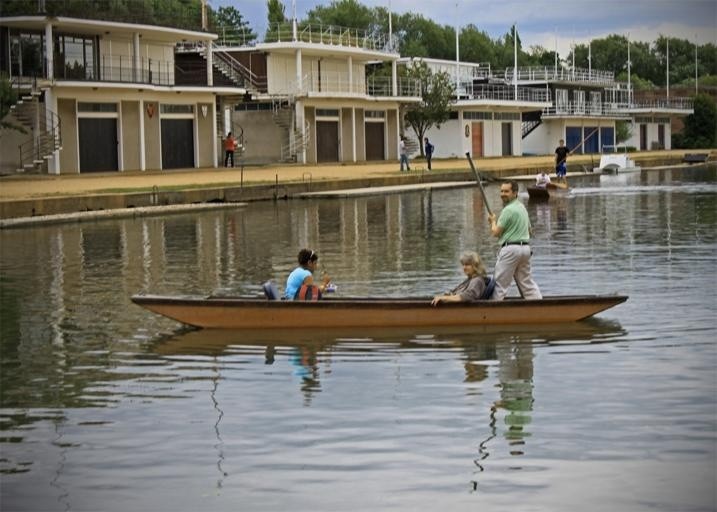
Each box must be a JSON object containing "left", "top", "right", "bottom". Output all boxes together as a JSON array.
[
  {"left": 264, "top": 280, "right": 283, "bottom": 301},
  {"left": 481, "top": 274, "right": 496, "bottom": 299}
]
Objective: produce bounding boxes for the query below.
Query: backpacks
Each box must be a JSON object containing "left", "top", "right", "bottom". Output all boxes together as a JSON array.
[{"left": 293, "top": 285, "right": 322, "bottom": 300}]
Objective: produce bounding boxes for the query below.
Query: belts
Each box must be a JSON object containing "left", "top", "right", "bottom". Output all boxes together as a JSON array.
[{"left": 502, "top": 241, "right": 528, "bottom": 247}]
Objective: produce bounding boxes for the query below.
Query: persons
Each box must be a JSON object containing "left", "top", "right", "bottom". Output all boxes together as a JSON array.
[
  {"left": 288, "top": 344, "right": 323, "bottom": 406},
  {"left": 423, "top": 137, "right": 434, "bottom": 171},
  {"left": 222, "top": 131, "right": 236, "bottom": 169},
  {"left": 553, "top": 139, "right": 573, "bottom": 187},
  {"left": 534, "top": 170, "right": 551, "bottom": 189},
  {"left": 445, "top": 336, "right": 489, "bottom": 383},
  {"left": 398, "top": 137, "right": 411, "bottom": 172},
  {"left": 429, "top": 249, "right": 488, "bottom": 309},
  {"left": 284, "top": 246, "right": 330, "bottom": 301},
  {"left": 486, "top": 178, "right": 545, "bottom": 301},
  {"left": 490, "top": 336, "right": 535, "bottom": 456}
]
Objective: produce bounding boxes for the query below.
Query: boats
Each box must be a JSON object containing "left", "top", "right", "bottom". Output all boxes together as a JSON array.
[
  {"left": 527, "top": 181, "right": 567, "bottom": 204},
  {"left": 129, "top": 276, "right": 628, "bottom": 328}
]
